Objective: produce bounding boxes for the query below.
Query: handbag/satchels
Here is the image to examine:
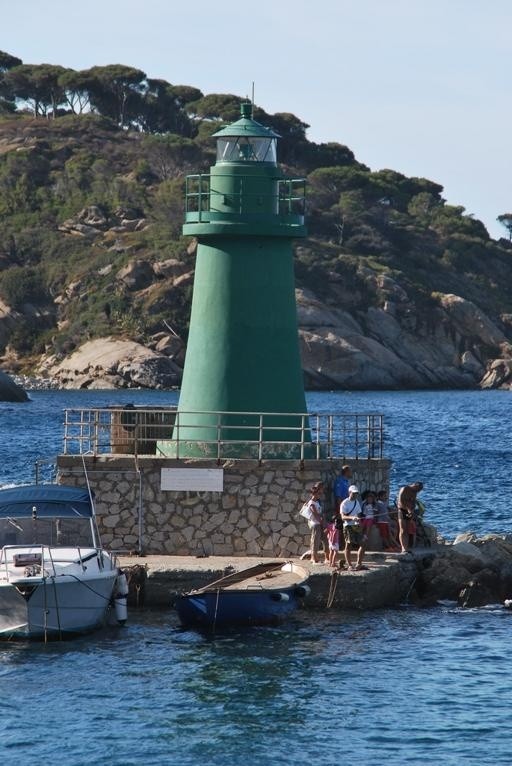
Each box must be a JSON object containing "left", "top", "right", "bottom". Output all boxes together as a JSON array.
[
  {"left": 299, "top": 504, "right": 313, "bottom": 519},
  {"left": 335, "top": 519, "right": 343, "bottom": 529}
]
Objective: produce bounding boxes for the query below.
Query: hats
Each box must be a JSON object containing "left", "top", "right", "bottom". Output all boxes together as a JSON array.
[{"left": 348, "top": 485, "right": 358, "bottom": 493}]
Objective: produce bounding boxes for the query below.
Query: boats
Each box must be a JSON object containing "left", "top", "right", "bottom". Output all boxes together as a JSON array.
[
  {"left": 0, "top": 459, "right": 132, "bottom": 641},
  {"left": 173, "top": 560, "right": 312, "bottom": 634}
]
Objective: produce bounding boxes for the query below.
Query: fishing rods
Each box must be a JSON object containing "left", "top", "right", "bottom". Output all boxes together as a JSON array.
[{"left": 359, "top": 511, "right": 401, "bottom": 520}]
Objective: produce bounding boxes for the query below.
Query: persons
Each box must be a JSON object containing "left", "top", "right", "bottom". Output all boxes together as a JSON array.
[{"left": 305, "top": 464, "right": 425, "bottom": 571}]
